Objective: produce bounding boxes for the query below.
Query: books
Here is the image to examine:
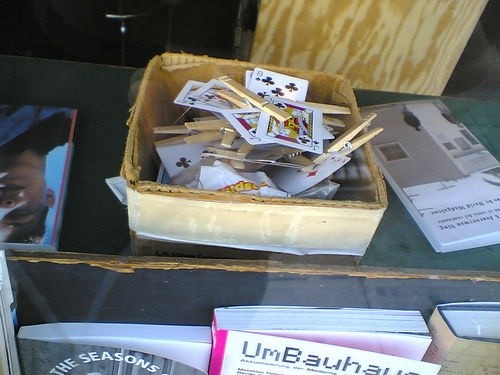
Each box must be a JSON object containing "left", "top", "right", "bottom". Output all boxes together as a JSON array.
[
  {"left": 358, "top": 98, "right": 500, "bottom": 255},
  {"left": 422, "top": 300, "right": 500, "bottom": 374},
  {"left": 14, "top": 322, "right": 212, "bottom": 375},
  {"left": 208, "top": 304, "right": 442, "bottom": 374}
]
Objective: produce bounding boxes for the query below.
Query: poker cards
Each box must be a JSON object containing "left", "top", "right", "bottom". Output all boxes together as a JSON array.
[{"left": 154, "top": 68, "right": 352, "bottom": 196}]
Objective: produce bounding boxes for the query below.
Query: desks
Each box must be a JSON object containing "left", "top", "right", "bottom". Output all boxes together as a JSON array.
[{"left": 0, "top": 53, "right": 500, "bottom": 375}]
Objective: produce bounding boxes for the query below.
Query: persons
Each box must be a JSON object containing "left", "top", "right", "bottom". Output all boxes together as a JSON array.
[{"left": 0, "top": 104, "right": 71, "bottom": 246}]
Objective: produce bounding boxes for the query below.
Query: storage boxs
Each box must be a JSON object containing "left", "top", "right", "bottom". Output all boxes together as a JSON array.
[{"left": 119, "top": 52, "right": 390, "bottom": 257}]
